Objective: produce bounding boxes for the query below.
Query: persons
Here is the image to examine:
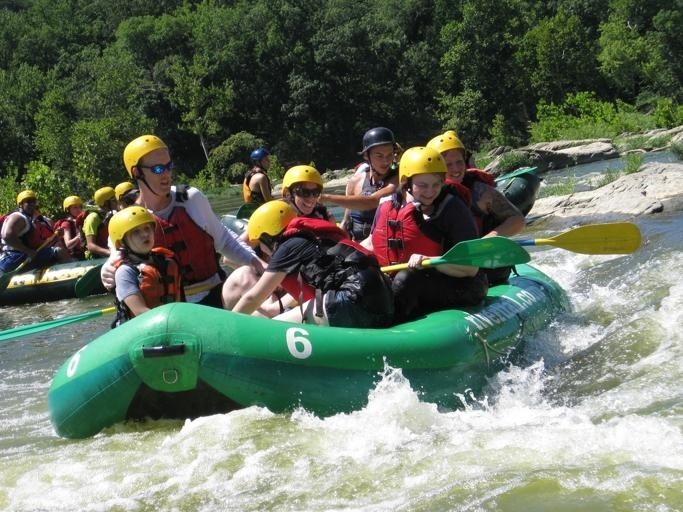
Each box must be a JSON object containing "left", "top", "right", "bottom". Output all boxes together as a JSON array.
[
  {"left": 0, "top": 182, "right": 139, "bottom": 274},
  {"left": 108, "top": 206, "right": 181, "bottom": 329},
  {"left": 224, "top": 164, "right": 394, "bottom": 329},
  {"left": 240, "top": 149, "right": 272, "bottom": 202},
  {"left": 339, "top": 128, "right": 524, "bottom": 313},
  {"left": 99, "top": 135, "right": 278, "bottom": 316}
]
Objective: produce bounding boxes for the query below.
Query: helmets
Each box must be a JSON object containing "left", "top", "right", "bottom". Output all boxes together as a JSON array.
[
  {"left": 63, "top": 196, "right": 81, "bottom": 212},
  {"left": 356, "top": 127, "right": 395, "bottom": 155},
  {"left": 250, "top": 148, "right": 267, "bottom": 160},
  {"left": 107, "top": 205, "right": 156, "bottom": 249},
  {"left": 399, "top": 131, "right": 465, "bottom": 186},
  {"left": 282, "top": 165, "right": 324, "bottom": 198},
  {"left": 16, "top": 190, "right": 36, "bottom": 207},
  {"left": 123, "top": 135, "right": 169, "bottom": 178},
  {"left": 94, "top": 186, "right": 114, "bottom": 205},
  {"left": 114, "top": 182, "right": 134, "bottom": 201},
  {"left": 248, "top": 201, "right": 295, "bottom": 242}
]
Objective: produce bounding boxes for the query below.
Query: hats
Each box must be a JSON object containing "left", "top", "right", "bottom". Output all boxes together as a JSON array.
[{"left": 123, "top": 189, "right": 140, "bottom": 199}]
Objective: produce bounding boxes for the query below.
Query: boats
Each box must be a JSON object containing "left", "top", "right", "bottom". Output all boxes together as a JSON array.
[
  {"left": 0, "top": 250, "right": 222, "bottom": 307},
  {"left": 46, "top": 261, "right": 574, "bottom": 442},
  {"left": 220, "top": 167, "right": 544, "bottom": 237}
]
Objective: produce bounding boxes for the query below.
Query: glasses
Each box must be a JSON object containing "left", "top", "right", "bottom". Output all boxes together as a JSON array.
[
  {"left": 140, "top": 159, "right": 174, "bottom": 173},
  {"left": 291, "top": 186, "right": 321, "bottom": 198}
]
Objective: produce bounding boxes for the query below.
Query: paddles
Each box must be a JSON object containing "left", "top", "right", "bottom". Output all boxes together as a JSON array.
[
  {"left": 0, "top": 225, "right": 61, "bottom": 287},
  {"left": 512, "top": 221, "right": 641, "bottom": 254},
  {"left": 236, "top": 202, "right": 260, "bottom": 218},
  {"left": 376, "top": 235, "right": 531, "bottom": 272},
  {"left": 0, "top": 281, "right": 220, "bottom": 342},
  {"left": 493, "top": 166, "right": 537, "bottom": 182},
  {"left": 75, "top": 264, "right": 103, "bottom": 298}
]
